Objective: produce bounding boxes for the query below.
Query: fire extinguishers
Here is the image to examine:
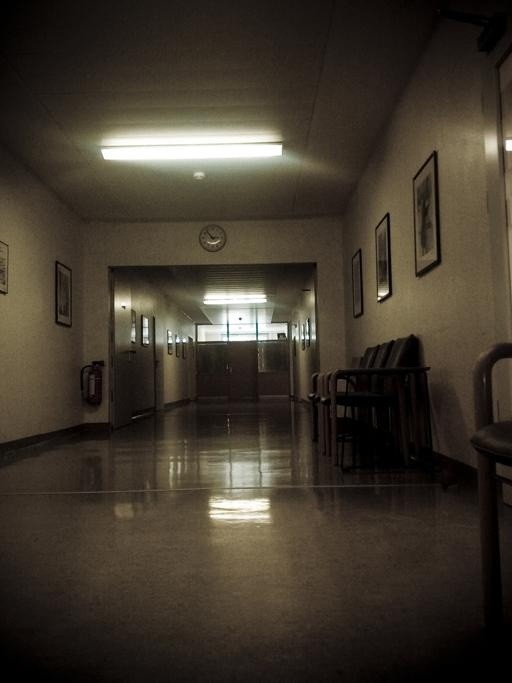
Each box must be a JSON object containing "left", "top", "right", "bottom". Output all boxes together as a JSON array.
[{"left": 80, "top": 361, "right": 105, "bottom": 405}]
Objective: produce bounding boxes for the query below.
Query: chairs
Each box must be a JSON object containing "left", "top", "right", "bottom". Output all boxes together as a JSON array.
[
  {"left": 468, "top": 343, "right": 512, "bottom": 633},
  {"left": 309, "top": 332, "right": 431, "bottom": 468}
]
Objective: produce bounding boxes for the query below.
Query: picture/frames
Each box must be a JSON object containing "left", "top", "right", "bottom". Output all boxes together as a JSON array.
[
  {"left": 350, "top": 246, "right": 364, "bottom": 320},
  {"left": 54, "top": 258, "right": 74, "bottom": 328},
  {"left": 300, "top": 317, "right": 310, "bottom": 350},
  {"left": 374, "top": 209, "right": 393, "bottom": 305},
  {"left": 141, "top": 314, "right": 150, "bottom": 346},
  {"left": 165, "top": 327, "right": 188, "bottom": 361},
  {"left": 130, "top": 306, "right": 137, "bottom": 345},
  {"left": 410, "top": 147, "right": 441, "bottom": 279},
  {"left": 0, "top": 239, "right": 10, "bottom": 295}
]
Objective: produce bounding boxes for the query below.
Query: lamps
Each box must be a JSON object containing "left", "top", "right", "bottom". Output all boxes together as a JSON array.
[{"left": 98, "top": 125, "right": 286, "bottom": 163}]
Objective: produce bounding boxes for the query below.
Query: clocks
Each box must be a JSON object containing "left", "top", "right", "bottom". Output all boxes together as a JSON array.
[{"left": 197, "top": 221, "right": 229, "bottom": 253}]
